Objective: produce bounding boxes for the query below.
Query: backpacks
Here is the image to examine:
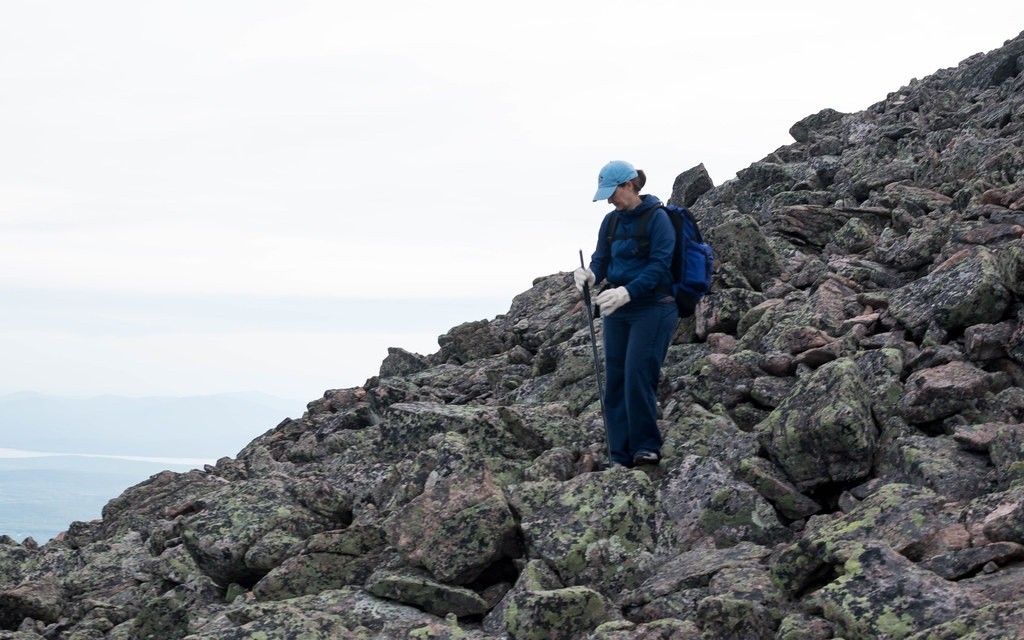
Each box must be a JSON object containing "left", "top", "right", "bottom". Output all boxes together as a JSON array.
[{"left": 605, "top": 203, "right": 714, "bottom": 318}]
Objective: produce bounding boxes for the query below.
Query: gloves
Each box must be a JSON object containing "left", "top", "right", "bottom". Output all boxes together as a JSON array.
[
  {"left": 574, "top": 266, "right": 596, "bottom": 294},
  {"left": 596, "top": 286, "right": 631, "bottom": 316}
]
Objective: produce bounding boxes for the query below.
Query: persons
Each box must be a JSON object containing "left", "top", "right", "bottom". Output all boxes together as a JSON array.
[{"left": 573, "top": 160, "right": 679, "bottom": 471}]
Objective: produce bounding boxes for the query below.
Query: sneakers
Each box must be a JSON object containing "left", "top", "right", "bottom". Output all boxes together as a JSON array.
[
  {"left": 599, "top": 456, "right": 624, "bottom": 470},
  {"left": 633, "top": 448, "right": 659, "bottom": 463}
]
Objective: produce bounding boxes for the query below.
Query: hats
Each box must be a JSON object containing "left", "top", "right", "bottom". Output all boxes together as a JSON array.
[{"left": 593, "top": 160, "right": 638, "bottom": 202}]
